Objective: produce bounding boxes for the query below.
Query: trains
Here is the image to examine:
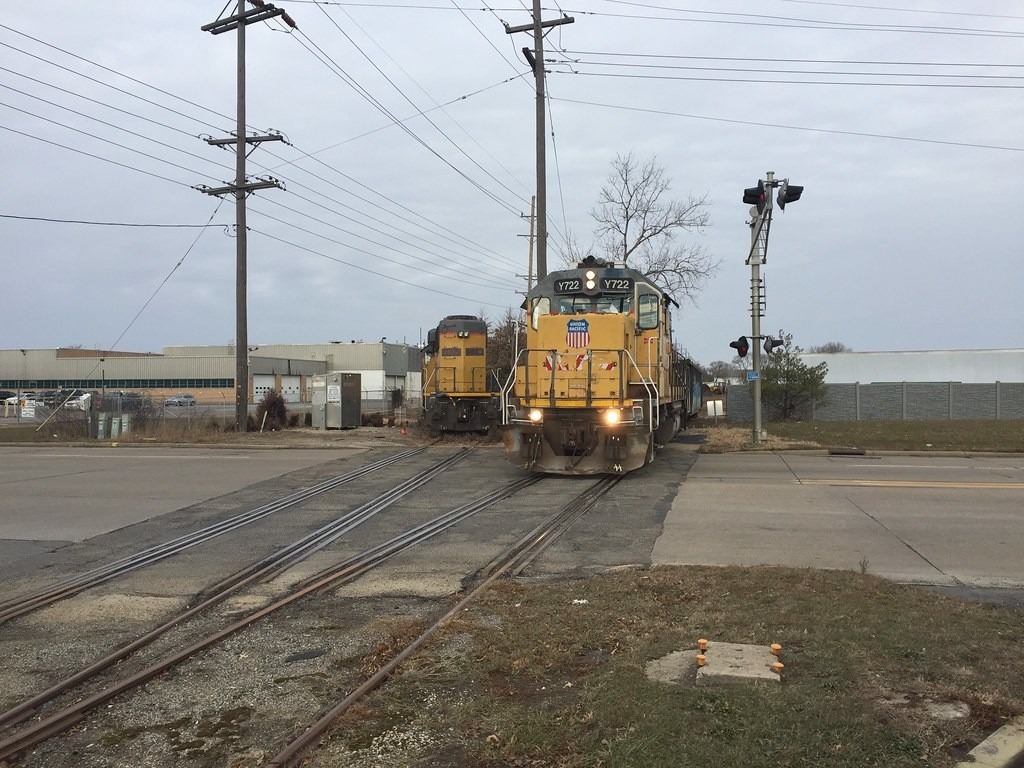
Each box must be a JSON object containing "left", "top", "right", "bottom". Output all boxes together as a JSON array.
[
  {"left": 417, "top": 313, "right": 496, "bottom": 436},
  {"left": 502, "top": 255, "right": 702, "bottom": 479}
]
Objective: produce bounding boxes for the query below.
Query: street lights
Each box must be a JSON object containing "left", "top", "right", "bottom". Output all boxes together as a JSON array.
[{"left": 99, "top": 358, "right": 106, "bottom": 396}]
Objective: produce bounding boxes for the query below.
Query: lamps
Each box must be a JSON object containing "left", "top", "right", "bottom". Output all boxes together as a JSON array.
[{"left": 20, "top": 349, "right": 25, "bottom": 355}]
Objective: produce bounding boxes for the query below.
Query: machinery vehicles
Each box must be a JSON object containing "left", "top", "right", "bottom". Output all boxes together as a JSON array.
[{"left": 708, "top": 376, "right": 726, "bottom": 393}]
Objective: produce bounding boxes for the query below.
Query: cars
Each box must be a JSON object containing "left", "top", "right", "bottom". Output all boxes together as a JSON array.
[
  {"left": 164, "top": 394, "right": 196, "bottom": 407},
  {"left": 0, "top": 390, "right": 143, "bottom": 411}
]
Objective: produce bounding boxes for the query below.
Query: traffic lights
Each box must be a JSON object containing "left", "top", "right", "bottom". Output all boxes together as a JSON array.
[
  {"left": 742, "top": 180, "right": 766, "bottom": 214},
  {"left": 775, "top": 179, "right": 805, "bottom": 210},
  {"left": 765, "top": 336, "right": 786, "bottom": 355},
  {"left": 730, "top": 336, "right": 749, "bottom": 357}
]
